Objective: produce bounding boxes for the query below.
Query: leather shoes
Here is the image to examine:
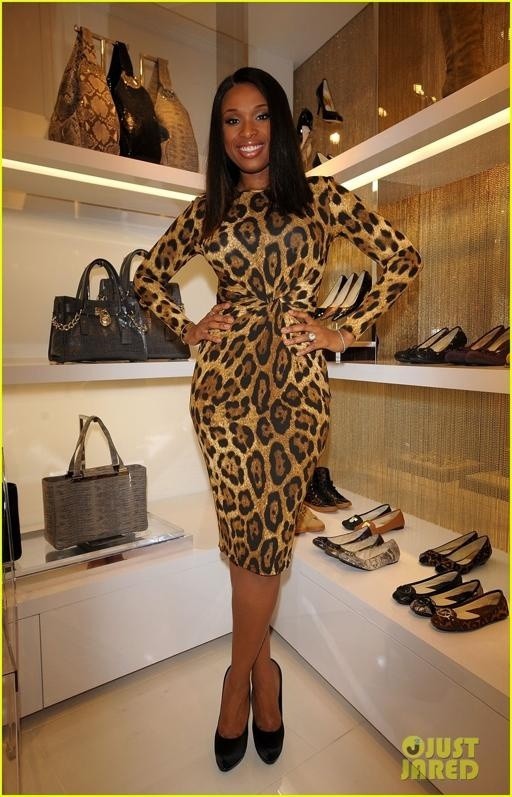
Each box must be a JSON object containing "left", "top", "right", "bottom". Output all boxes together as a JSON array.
[
  {"left": 432, "top": 590, "right": 509, "bottom": 632},
  {"left": 343, "top": 504, "right": 391, "bottom": 530},
  {"left": 326, "top": 533, "right": 383, "bottom": 559},
  {"left": 435, "top": 535, "right": 492, "bottom": 574},
  {"left": 410, "top": 580, "right": 484, "bottom": 618},
  {"left": 312, "top": 527, "right": 372, "bottom": 550},
  {"left": 338, "top": 540, "right": 400, "bottom": 571},
  {"left": 392, "top": 570, "right": 462, "bottom": 605},
  {"left": 354, "top": 510, "right": 406, "bottom": 534},
  {"left": 419, "top": 532, "right": 478, "bottom": 566}
]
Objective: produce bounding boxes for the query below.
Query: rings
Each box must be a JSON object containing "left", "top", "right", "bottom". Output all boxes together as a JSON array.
[
  {"left": 209, "top": 308, "right": 217, "bottom": 316},
  {"left": 308, "top": 333, "right": 316, "bottom": 342}
]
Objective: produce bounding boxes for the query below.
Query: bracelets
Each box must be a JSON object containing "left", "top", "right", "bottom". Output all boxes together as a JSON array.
[{"left": 338, "top": 328, "right": 346, "bottom": 352}]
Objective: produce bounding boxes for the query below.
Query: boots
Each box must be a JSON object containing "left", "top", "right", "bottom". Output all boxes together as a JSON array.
[
  {"left": 302, "top": 467, "right": 337, "bottom": 512},
  {"left": 312, "top": 466, "right": 352, "bottom": 509}
]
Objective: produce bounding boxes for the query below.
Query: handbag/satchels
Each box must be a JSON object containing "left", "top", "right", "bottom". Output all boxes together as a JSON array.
[
  {"left": 42, "top": 416, "right": 147, "bottom": 550},
  {"left": 107, "top": 40, "right": 169, "bottom": 165},
  {"left": 146, "top": 53, "right": 201, "bottom": 173},
  {"left": 51, "top": 26, "right": 120, "bottom": 155},
  {"left": 46, "top": 259, "right": 147, "bottom": 361},
  {"left": 99, "top": 250, "right": 191, "bottom": 360},
  {"left": 3, "top": 481, "right": 22, "bottom": 562}
]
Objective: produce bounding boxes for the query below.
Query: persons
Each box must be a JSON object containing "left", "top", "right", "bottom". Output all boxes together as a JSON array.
[{"left": 131, "top": 64, "right": 424, "bottom": 773}]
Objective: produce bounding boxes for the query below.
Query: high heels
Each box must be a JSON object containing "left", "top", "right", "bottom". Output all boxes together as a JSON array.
[
  {"left": 320, "top": 273, "right": 359, "bottom": 321},
  {"left": 315, "top": 78, "right": 344, "bottom": 124},
  {"left": 445, "top": 324, "right": 505, "bottom": 364},
  {"left": 465, "top": 327, "right": 510, "bottom": 366},
  {"left": 407, "top": 326, "right": 467, "bottom": 364},
  {"left": 297, "top": 107, "right": 314, "bottom": 150},
  {"left": 214, "top": 662, "right": 250, "bottom": 771},
  {"left": 332, "top": 270, "right": 372, "bottom": 321},
  {"left": 311, "top": 152, "right": 334, "bottom": 168},
  {"left": 394, "top": 327, "right": 449, "bottom": 362},
  {"left": 252, "top": 659, "right": 284, "bottom": 766},
  {"left": 313, "top": 276, "right": 348, "bottom": 320}
]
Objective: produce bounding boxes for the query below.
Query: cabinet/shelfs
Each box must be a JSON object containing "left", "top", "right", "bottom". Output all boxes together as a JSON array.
[
  {"left": 3, "top": 128, "right": 232, "bottom": 727},
  {"left": 268, "top": 64, "right": 511, "bottom": 795}
]
